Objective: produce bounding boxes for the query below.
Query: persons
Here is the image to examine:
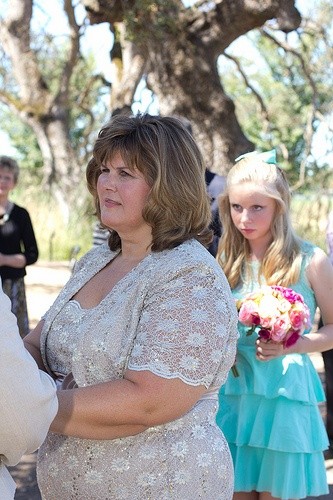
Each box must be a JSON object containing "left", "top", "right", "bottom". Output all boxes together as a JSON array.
[{"left": 0, "top": 115, "right": 333, "bottom": 500}]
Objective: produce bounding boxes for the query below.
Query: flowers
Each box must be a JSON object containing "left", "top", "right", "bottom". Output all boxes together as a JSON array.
[{"left": 235, "top": 285, "right": 310, "bottom": 355}]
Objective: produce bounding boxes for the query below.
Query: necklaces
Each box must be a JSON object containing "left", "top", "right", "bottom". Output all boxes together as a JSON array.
[{"left": 0, "top": 202, "right": 13, "bottom": 226}]
